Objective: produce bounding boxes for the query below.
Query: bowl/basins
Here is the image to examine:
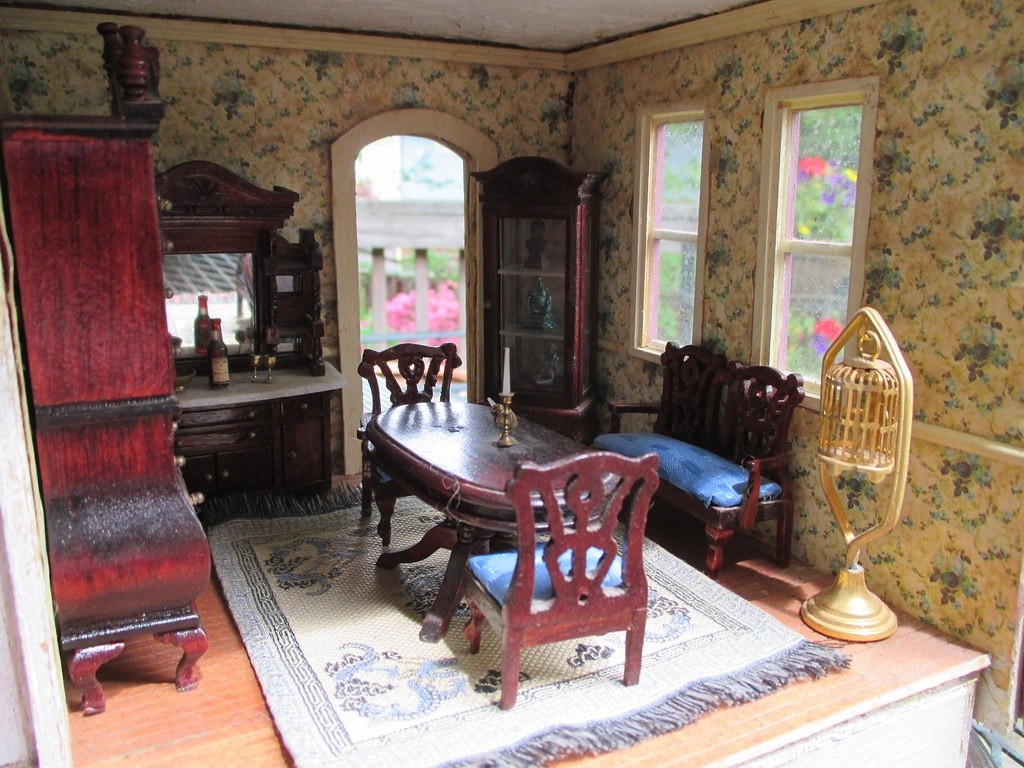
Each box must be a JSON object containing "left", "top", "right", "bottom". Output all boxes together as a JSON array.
[
  {"left": 173, "top": 366, "right": 196, "bottom": 393},
  {"left": 173, "top": 337, "right": 182, "bottom": 352}
]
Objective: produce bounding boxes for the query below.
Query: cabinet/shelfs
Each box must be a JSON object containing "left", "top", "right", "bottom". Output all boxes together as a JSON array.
[
  {"left": 1, "top": 113, "right": 210, "bottom": 716},
  {"left": 471, "top": 157, "right": 610, "bottom": 447},
  {"left": 152, "top": 158, "right": 345, "bottom": 498}
]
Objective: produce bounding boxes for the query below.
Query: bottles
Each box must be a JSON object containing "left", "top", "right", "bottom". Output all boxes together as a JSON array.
[
  {"left": 209, "top": 318, "right": 231, "bottom": 387},
  {"left": 195, "top": 295, "right": 212, "bottom": 356}
]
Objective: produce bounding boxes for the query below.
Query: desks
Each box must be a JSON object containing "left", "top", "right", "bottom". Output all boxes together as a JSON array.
[{"left": 365, "top": 400, "right": 622, "bottom": 644}]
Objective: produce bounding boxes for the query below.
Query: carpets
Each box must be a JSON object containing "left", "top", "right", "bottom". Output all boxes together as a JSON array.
[{"left": 195, "top": 477, "right": 852, "bottom": 768}]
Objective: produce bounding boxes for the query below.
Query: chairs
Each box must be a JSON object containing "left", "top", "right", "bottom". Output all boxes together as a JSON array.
[
  {"left": 357, "top": 344, "right": 463, "bottom": 537},
  {"left": 462, "top": 449, "right": 659, "bottom": 709}
]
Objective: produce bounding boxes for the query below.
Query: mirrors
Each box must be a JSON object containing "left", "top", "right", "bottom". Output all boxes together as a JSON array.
[{"left": 161, "top": 252, "right": 255, "bottom": 358}]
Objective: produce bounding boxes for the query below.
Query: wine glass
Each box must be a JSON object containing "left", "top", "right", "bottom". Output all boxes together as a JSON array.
[
  {"left": 250, "top": 354, "right": 262, "bottom": 383},
  {"left": 265, "top": 354, "right": 277, "bottom": 383},
  {"left": 236, "top": 330, "right": 245, "bottom": 355},
  {"left": 247, "top": 326, "right": 256, "bottom": 356}
]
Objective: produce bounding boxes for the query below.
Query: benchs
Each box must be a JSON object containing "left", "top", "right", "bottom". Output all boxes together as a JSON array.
[{"left": 590, "top": 341, "right": 807, "bottom": 579}]
